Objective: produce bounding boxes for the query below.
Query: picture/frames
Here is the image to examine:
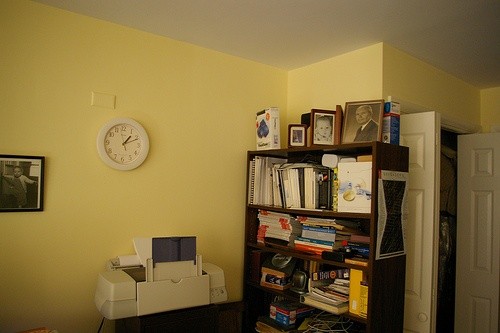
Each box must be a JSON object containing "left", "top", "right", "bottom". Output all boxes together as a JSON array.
[
  {"left": 0, "top": 153, "right": 46, "bottom": 212},
  {"left": 309, "top": 107, "right": 336, "bottom": 147},
  {"left": 286, "top": 122, "right": 308, "bottom": 147},
  {"left": 340, "top": 98, "right": 386, "bottom": 144}
]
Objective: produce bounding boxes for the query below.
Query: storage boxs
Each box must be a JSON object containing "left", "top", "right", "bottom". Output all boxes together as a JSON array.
[
  {"left": 348, "top": 268, "right": 367, "bottom": 316},
  {"left": 269, "top": 298, "right": 316, "bottom": 326},
  {"left": 381, "top": 101, "right": 401, "bottom": 145},
  {"left": 359, "top": 281, "right": 368, "bottom": 319},
  {"left": 260, "top": 266, "right": 292, "bottom": 291},
  {"left": 256, "top": 106, "right": 281, "bottom": 151}
]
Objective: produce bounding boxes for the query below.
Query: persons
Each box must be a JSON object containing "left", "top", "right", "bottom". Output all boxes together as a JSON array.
[
  {"left": 0, "top": 166, "right": 37, "bottom": 209},
  {"left": 353, "top": 105, "right": 379, "bottom": 141},
  {"left": 314, "top": 117, "right": 332, "bottom": 142}
]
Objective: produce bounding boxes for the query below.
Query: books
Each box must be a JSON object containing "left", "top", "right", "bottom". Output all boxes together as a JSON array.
[{"left": 248, "top": 156, "right": 372, "bottom": 318}]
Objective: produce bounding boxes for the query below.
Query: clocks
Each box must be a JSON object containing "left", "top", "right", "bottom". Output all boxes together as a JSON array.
[{"left": 95, "top": 116, "right": 151, "bottom": 172}]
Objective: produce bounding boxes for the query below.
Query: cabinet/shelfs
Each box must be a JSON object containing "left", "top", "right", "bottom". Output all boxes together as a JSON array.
[{"left": 239, "top": 142, "right": 410, "bottom": 333}]
